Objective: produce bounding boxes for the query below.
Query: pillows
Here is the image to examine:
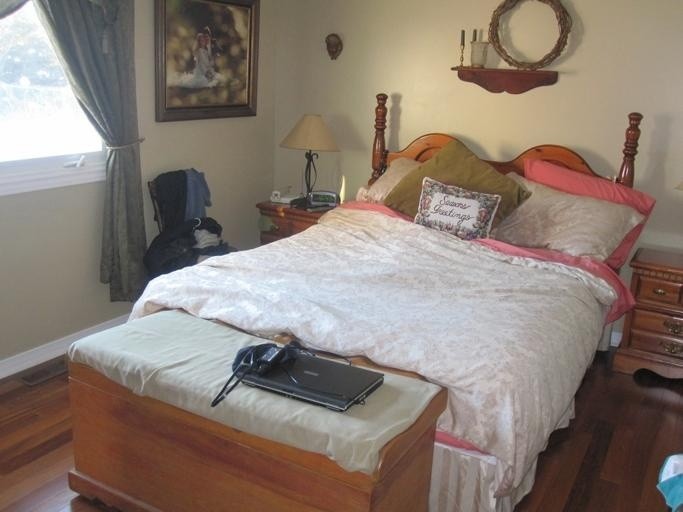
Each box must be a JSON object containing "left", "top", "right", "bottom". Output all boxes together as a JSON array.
[
  {"left": 414, "top": 177, "right": 501, "bottom": 240},
  {"left": 522, "top": 156, "right": 657, "bottom": 269},
  {"left": 370, "top": 157, "right": 423, "bottom": 202},
  {"left": 489, "top": 171, "right": 646, "bottom": 264},
  {"left": 384, "top": 139, "right": 533, "bottom": 234}
]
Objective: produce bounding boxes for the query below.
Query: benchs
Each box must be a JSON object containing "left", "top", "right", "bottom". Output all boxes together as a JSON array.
[{"left": 67, "top": 310, "right": 448, "bottom": 512}]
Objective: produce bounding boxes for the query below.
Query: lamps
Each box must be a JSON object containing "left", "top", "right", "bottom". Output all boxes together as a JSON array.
[{"left": 278, "top": 114, "right": 342, "bottom": 210}]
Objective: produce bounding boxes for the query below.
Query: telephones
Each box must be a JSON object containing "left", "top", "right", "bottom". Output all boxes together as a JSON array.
[{"left": 269, "top": 185, "right": 305, "bottom": 205}]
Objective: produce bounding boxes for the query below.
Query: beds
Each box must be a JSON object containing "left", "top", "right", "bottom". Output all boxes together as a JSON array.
[{"left": 126, "top": 93, "right": 643, "bottom": 512}]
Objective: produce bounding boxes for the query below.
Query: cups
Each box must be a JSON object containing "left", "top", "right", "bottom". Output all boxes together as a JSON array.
[{"left": 469, "top": 40, "right": 489, "bottom": 67}]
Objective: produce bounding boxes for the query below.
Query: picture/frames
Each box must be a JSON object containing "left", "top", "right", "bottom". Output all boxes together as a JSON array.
[{"left": 154, "top": 0, "right": 260, "bottom": 123}]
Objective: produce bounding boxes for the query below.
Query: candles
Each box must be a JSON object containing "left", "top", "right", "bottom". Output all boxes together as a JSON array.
[
  {"left": 461, "top": 30, "right": 465, "bottom": 45},
  {"left": 473, "top": 29, "right": 476, "bottom": 42}
]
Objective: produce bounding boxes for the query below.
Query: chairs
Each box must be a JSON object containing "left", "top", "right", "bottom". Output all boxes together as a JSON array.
[{"left": 147, "top": 169, "right": 237, "bottom": 263}]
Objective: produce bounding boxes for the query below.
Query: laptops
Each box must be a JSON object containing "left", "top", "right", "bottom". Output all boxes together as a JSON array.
[{"left": 233, "top": 342, "right": 385, "bottom": 412}]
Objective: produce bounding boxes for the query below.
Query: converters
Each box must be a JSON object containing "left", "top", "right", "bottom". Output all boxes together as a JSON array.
[{"left": 260, "top": 347, "right": 285, "bottom": 366}]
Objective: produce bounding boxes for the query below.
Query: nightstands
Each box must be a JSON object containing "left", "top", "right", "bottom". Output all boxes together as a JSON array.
[
  {"left": 611, "top": 247, "right": 683, "bottom": 377},
  {"left": 255, "top": 200, "right": 323, "bottom": 246}
]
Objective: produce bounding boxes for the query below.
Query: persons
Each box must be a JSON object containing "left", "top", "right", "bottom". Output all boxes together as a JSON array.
[
  {"left": 191, "top": 31, "right": 217, "bottom": 89},
  {"left": 201, "top": 18, "right": 225, "bottom": 62}
]
